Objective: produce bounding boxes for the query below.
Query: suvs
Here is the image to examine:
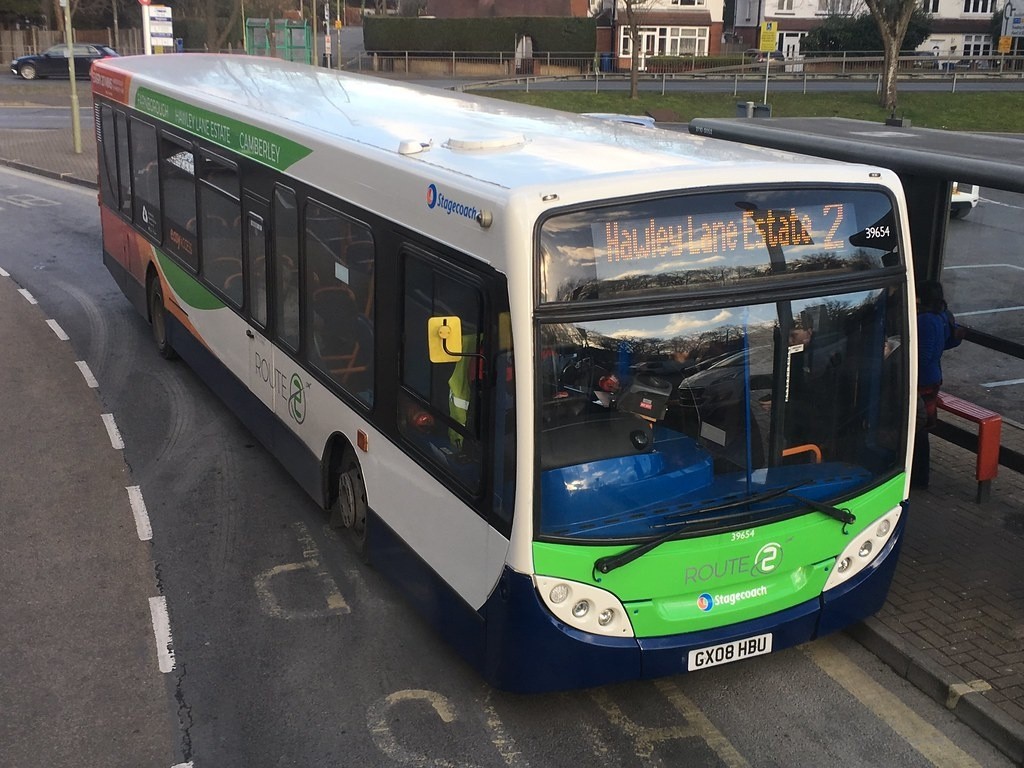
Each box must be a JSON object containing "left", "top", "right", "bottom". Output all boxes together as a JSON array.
[
  {"left": 744, "top": 49, "right": 784, "bottom": 62},
  {"left": 582, "top": 112, "right": 656, "bottom": 128}
]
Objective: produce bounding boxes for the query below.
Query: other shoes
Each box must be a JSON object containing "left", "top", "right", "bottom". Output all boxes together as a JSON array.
[{"left": 912, "top": 479, "right": 929, "bottom": 489}]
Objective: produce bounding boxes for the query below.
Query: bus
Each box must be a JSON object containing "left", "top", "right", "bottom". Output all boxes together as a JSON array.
[{"left": 89, "top": 52, "right": 920, "bottom": 691}]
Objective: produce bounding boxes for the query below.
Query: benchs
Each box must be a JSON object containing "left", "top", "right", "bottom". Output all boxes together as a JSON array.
[{"left": 937, "top": 389, "right": 1003, "bottom": 505}]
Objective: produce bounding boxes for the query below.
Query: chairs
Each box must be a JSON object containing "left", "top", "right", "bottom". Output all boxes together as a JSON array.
[{"left": 147, "top": 161, "right": 374, "bottom": 384}]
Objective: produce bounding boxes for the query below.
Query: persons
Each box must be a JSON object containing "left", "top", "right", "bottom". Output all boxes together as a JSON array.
[
  {"left": 469, "top": 339, "right": 618, "bottom": 431},
  {"left": 759, "top": 311, "right": 831, "bottom": 464},
  {"left": 910, "top": 279, "right": 962, "bottom": 489},
  {"left": 661, "top": 328, "right": 765, "bottom": 474}
]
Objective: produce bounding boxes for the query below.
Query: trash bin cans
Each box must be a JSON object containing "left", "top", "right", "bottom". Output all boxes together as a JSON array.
[
  {"left": 600, "top": 53, "right": 614, "bottom": 71},
  {"left": 736, "top": 102, "right": 773, "bottom": 118},
  {"left": 943, "top": 63, "right": 957, "bottom": 70},
  {"left": 176, "top": 38, "right": 184, "bottom": 50}
]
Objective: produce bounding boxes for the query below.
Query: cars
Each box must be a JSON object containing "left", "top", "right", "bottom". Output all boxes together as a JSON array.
[
  {"left": 949, "top": 181, "right": 981, "bottom": 219},
  {"left": 677, "top": 344, "right": 774, "bottom": 412},
  {"left": 10, "top": 43, "right": 122, "bottom": 81}
]
